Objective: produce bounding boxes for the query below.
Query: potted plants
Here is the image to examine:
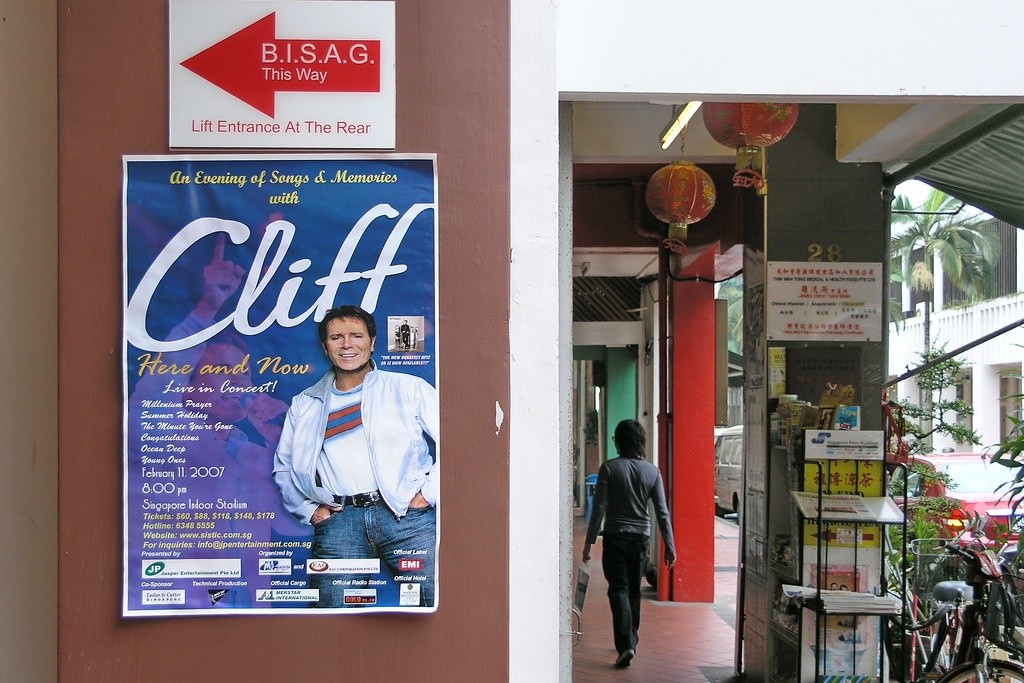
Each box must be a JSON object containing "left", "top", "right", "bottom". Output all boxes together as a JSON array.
[{"left": 884, "top": 506, "right": 942, "bottom": 683}]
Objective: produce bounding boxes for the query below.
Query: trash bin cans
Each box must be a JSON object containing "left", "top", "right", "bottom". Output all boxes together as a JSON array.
[{"left": 585, "top": 474, "right": 599, "bottom": 522}]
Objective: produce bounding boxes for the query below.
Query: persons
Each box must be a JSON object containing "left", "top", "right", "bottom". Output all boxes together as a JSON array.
[
  {"left": 128, "top": 231, "right": 315, "bottom": 610},
  {"left": 272, "top": 305, "right": 436, "bottom": 608},
  {"left": 401, "top": 320, "right": 410, "bottom": 349},
  {"left": 582, "top": 419, "right": 676, "bottom": 667}
]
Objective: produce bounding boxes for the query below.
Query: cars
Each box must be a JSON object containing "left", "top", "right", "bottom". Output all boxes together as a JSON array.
[{"left": 887, "top": 452, "right": 1024, "bottom": 552}]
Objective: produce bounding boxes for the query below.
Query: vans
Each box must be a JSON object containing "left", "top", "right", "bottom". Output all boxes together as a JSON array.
[{"left": 714, "top": 425, "right": 743, "bottom": 525}]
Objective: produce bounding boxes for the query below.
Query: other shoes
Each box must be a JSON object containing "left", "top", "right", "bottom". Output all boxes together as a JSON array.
[{"left": 615, "top": 648, "right": 635, "bottom": 667}]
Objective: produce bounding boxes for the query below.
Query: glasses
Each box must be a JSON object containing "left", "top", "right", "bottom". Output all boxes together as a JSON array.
[{"left": 612, "top": 437, "right": 616, "bottom": 441}]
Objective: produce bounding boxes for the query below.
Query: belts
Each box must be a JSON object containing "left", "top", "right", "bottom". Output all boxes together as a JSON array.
[{"left": 332, "top": 490, "right": 380, "bottom": 506}]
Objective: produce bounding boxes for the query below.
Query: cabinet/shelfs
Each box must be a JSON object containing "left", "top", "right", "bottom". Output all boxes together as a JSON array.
[
  {"left": 791, "top": 426, "right": 909, "bottom": 683},
  {"left": 769, "top": 444, "right": 799, "bottom": 683}
]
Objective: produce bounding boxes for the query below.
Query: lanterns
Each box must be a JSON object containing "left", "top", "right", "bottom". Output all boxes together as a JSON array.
[
  {"left": 646, "top": 160, "right": 716, "bottom": 238},
  {"left": 702, "top": 102, "right": 799, "bottom": 170}
]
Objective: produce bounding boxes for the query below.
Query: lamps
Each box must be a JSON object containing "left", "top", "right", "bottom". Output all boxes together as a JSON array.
[{"left": 660, "top": 101, "right": 704, "bottom": 151}]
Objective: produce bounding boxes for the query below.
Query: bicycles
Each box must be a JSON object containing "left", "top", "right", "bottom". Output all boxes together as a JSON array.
[{"left": 911, "top": 529, "right": 1024, "bottom": 683}]
